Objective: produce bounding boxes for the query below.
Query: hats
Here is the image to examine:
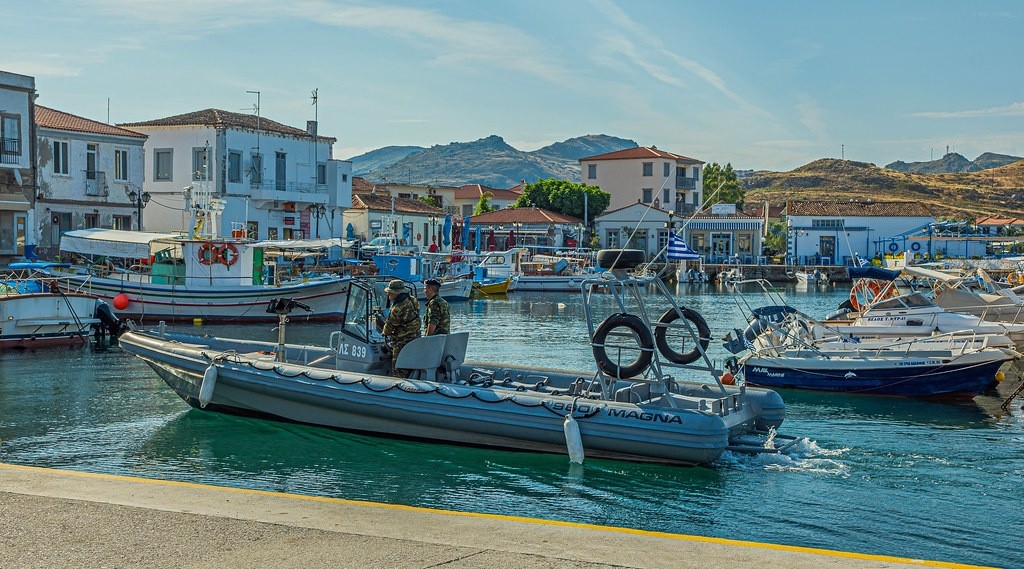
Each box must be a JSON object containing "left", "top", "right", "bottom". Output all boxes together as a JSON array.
[
  {"left": 424, "top": 280, "right": 441, "bottom": 288},
  {"left": 384, "top": 280, "right": 411, "bottom": 294}
]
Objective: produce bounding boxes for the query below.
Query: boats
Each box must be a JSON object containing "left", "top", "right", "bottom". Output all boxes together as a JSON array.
[
  {"left": 116, "top": 164, "right": 805, "bottom": 467},
  {"left": 27, "top": 139, "right": 357, "bottom": 326},
  {"left": 795, "top": 270, "right": 835, "bottom": 284},
  {"left": 263, "top": 239, "right": 653, "bottom": 302},
  {"left": 808, "top": 206, "right": 1024, "bottom": 394},
  {"left": 722, "top": 279, "right": 1014, "bottom": 401},
  {"left": 0, "top": 262, "right": 102, "bottom": 349},
  {"left": 676, "top": 255, "right": 706, "bottom": 283},
  {"left": 716, "top": 253, "right": 745, "bottom": 283}
]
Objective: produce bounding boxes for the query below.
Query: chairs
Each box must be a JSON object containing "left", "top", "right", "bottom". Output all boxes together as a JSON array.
[
  {"left": 792, "top": 256, "right": 801, "bottom": 265},
  {"left": 394, "top": 332, "right": 469, "bottom": 381}
]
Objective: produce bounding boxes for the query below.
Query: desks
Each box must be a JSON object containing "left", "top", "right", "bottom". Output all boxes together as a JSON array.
[
  {"left": 756, "top": 255, "right": 768, "bottom": 265},
  {"left": 822, "top": 257, "right": 831, "bottom": 266},
  {"left": 807, "top": 257, "right": 816, "bottom": 265}
]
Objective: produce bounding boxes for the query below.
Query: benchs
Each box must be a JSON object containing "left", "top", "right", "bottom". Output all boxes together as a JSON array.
[
  {"left": 729, "top": 256, "right": 738, "bottom": 264},
  {"left": 843, "top": 256, "right": 858, "bottom": 266}
]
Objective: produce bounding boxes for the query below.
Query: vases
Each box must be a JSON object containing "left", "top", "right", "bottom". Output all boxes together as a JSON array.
[{"left": 924, "top": 255, "right": 930, "bottom": 258}]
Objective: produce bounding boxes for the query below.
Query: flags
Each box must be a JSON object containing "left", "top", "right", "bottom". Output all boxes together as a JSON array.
[{"left": 667, "top": 231, "right": 700, "bottom": 260}]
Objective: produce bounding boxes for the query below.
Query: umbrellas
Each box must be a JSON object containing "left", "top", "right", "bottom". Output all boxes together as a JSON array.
[{"left": 442, "top": 214, "right": 481, "bottom": 267}]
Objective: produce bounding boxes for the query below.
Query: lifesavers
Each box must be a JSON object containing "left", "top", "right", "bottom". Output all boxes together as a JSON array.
[
  {"left": 889, "top": 243, "right": 898, "bottom": 251},
  {"left": 592, "top": 313, "right": 653, "bottom": 379},
  {"left": 655, "top": 307, "right": 711, "bottom": 364},
  {"left": 934, "top": 284, "right": 942, "bottom": 295},
  {"left": 198, "top": 243, "right": 218, "bottom": 264},
  {"left": 218, "top": 244, "right": 238, "bottom": 265},
  {"left": 1008, "top": 273, "right": 1019, "bottom": 284},
  {"left": 1020, "top": 274, "right": 1024, "bottom": 284},
  {"left": 850, "top": 281, "right": 883, "bottom": 311},
  {"left": 911, "top": 242, "right": 920, "bottom": 251},
  {"left": 879, "top": 281, "right": 893, "bottom": 299}
]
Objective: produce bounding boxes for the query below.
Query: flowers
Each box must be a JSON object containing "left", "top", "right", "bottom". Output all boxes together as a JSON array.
[{"left": 925, "top": 251, "right": 929, "bottom": 255}]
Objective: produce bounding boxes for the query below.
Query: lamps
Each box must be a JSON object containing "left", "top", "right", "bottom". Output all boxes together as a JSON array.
[
  {"left": 132, "top": 211, "right": 137, "bottom": 216},
  {"left": 46, "top": 206, "right": 51, "bottom": 213},
  {"left": 93, "top": 208, "right": 100, "bottom": 215}
]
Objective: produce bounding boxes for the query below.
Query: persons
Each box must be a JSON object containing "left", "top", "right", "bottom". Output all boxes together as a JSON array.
[
  {"left": 428, "top": 242, "right": 439, "bottom": 253},
  {"left": 381, "top": 279, "right": 421, "bottom": 379},
  {"left": 423, "top": 278, "right": 451, "bottom": 336}
]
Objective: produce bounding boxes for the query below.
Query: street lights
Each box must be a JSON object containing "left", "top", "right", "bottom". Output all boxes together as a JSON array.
[
  {"left": 128, "top": 189, "right": 151, "bottom": 232},
  {"left": 309, "top": 203, "right": 327, "bottom": 239},
  {"left": 789, "top": 227, "right": 805, "bottom": 266},
  {"left": 428, "top": 213, "right": 440, "bottom": 235},
  {"left": 512, "top": 220, "right": 523, "bottom": 248},
  {"left": 923, "top": 226, "right": 940, "bottom": 259},
  {"left": 669, "top": 210, "right": 674, "bottom": 231}
]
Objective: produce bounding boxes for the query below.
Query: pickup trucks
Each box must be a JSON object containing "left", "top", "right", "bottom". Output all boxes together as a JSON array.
[{"left": 359, "top": 237, "right": 420, "bottom": 264}]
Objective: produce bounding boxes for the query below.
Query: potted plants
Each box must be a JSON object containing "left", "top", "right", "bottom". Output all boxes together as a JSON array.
[
  {"left": 916, "top": 252, "right": 920, "bottom": 258},
  {"left": 936, "top": 252, "right": 945, "bottom": 259}
]
